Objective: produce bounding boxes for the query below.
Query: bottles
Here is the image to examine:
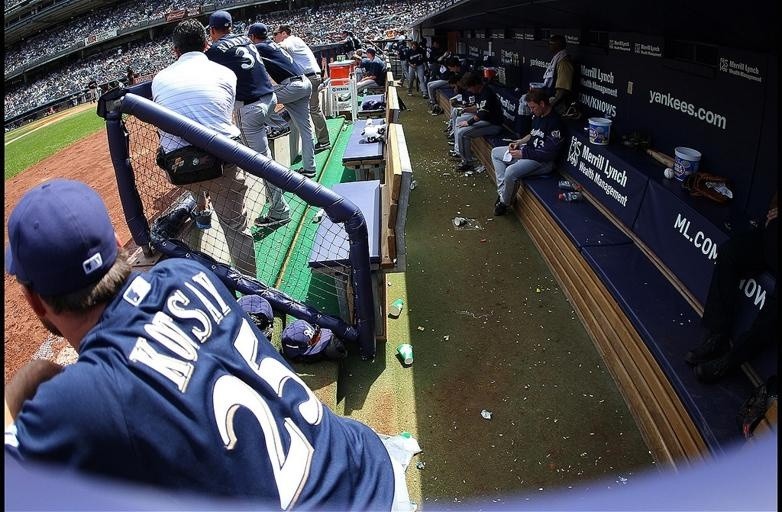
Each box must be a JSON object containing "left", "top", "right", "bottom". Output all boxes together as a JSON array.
[{"left": 558, "top": 192, "right": 582, "bottom": 202}]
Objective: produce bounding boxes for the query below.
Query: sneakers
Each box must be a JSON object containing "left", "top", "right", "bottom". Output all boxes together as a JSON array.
[
  {"left": 493, "top": 196, "right": 517, "bottom": 216},
  {"left": 314, "top": 143, "right": 332, "bottom": 150},
  {"left": 295, "top": 168, "right": 317, "bottom": 177},
  {"left": 266, "top": 128, "right": 291, "bottom": 139},
  {"left": 254, "top": 215, "right": 291, "bottom": 227},
  {"left": 189, "top": 200, "right": 211, "bottom": 229},
  {"left": 444, "top": 126, "right": 474, "bottom": 172}
]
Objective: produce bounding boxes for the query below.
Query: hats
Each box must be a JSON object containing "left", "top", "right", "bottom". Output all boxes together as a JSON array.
[
  {"left": 248, "top": 22, "right": 267, "bottom": 36},
  {"left": 5, "top": 179, "right": 117, "bottom": 290},
  {"left": 367, "top": 48, "right": 375, "bottom": 53},
  {"left": 362, "top": 101, "right": 381, "bottom": 110},
  {"left": 237, "top": 296, "right": 274, "bottom": 341},
  {"left": 204, "top": 9, "right": 232, "bottom": 29},
  {"left": 542, "top": 34, "right": 566, "bottom": 46},
  {"left": 281, "top": 322, "right": 331, "bottom": 357}
]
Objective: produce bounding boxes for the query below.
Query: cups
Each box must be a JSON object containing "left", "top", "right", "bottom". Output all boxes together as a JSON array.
[
  {"left": 395, "top": 344, "right": 414, "bottom": 365},
  {"left": 454, "top": 217, "right": 467, "bottom": 226},
  {"left": 388, "top": 299, "right": 404, "bottom": 317}
]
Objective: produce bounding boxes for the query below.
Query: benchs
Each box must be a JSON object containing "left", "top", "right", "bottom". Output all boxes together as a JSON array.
[{"left": 306, "top": 50, "right": 413, "bottom": 335}]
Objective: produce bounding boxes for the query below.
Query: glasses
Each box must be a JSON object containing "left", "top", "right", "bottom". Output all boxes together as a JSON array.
[{"left": 273, "top": 31, "right": 282, "bottom": 36}]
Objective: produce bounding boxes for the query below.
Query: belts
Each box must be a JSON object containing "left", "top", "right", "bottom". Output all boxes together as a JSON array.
[
  {"left": 289, "top": 76, "right": 302, "bottom": 82},
  {"left": 305, "top": 71, "right": 322, "bottom": 78}
]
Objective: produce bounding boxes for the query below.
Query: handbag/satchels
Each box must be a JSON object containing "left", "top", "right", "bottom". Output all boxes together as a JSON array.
[{"left": 158, "top": 140, "right": 222, "bottom": 182}]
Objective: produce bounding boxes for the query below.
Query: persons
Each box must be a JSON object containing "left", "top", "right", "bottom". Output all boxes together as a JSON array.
[
  {"left": 201, "top": 8, "right": 293, "bottom": 230},
  {"left": 543, "top": 33, "right": 576, "bottom": 118},
  {"left": 1, "top": 175, "right": 396, "bottom": 511},
  {"left": 680, "top": 186, "right": 780, "bottom": 387},
  {"left": 491, "top": 86, "right": 567, "bottom": 217},
  {"left": 245, "top": 23, "right": 318, "bottom": 180},
  {"left": 449, "top": 74, "right": 506, "bottom": 172},
  {"left": 149, "top": 15, "right": 258, "bottom": 288},
  {"left": 4, "top": 1, "right": 467, "bottom": 114}
]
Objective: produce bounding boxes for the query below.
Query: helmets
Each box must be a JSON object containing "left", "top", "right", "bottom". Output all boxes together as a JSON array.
[{"left": 554, "top": 97, "right": 588, "bottom": 122}]
